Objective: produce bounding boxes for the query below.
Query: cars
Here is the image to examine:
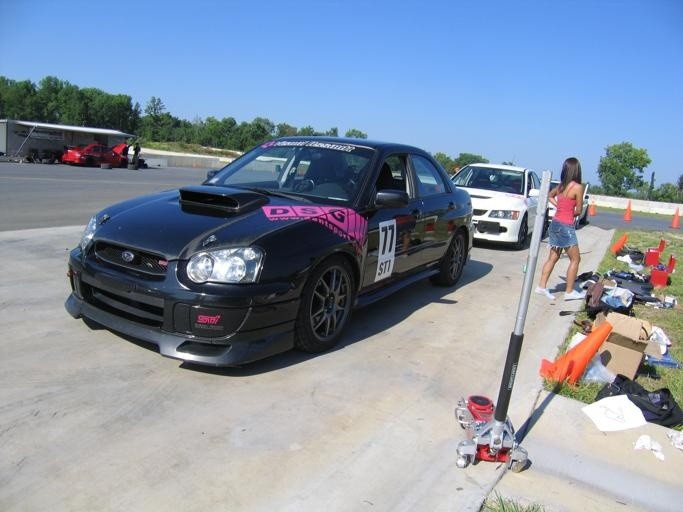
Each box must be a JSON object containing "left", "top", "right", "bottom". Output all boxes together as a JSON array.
[
  {"left": 61, "top": 143, "right": 129, "bottom": 166},
  {"left": 450, "top": 163, "right": 588, "bottom": 247},
  {"left": 65, "top": 135, "right": 474, "bottom": 367}
]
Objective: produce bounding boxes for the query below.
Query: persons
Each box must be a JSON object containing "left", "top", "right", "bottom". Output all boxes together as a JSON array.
[
  {"left": 130, "top": 143, "right": 141, "bottom": 164},
  {"left": 536, "top": 158, "right": 584, "bottom": 302}
]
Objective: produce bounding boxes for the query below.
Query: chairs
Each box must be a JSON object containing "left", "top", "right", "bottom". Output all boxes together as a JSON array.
[
  {"left": 357, "top": 158, "right": 395, "bottom": 186},
  {"left": 303, "top": 160, "right": 337, "bottom": 182}
]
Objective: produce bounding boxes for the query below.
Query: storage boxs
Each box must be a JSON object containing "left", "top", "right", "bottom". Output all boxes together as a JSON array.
[{"left": 592, "top": 311, "right": 663, "bottom": 382}]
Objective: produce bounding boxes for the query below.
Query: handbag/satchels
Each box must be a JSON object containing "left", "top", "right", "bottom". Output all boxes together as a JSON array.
[{"left": 595, "top": 374, "right": 683, "bottom": 426}]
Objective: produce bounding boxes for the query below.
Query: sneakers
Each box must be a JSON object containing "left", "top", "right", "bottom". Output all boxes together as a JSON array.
[
  {"left": 535, "top": 286, "right": 555, "bottom": 300},
  {"left": 565, "top": 290, "right": 585, "bottom": 300}
]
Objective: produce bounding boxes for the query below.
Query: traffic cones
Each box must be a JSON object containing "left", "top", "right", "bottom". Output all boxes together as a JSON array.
[
  {"left": 670, "top": 207, "right": 680, "bottom": 229},
  {"left": 589, "top": 201, "right": 595, "bottom": 216},
  {"left": 539, "top": 322, "right": 613, "bottom": 391},
  {"left": 624, "top": 201, "right": 632, "bottom": 221},
  {"left": 610, "top": 234, "right": 628, "bottom": 253}
]
